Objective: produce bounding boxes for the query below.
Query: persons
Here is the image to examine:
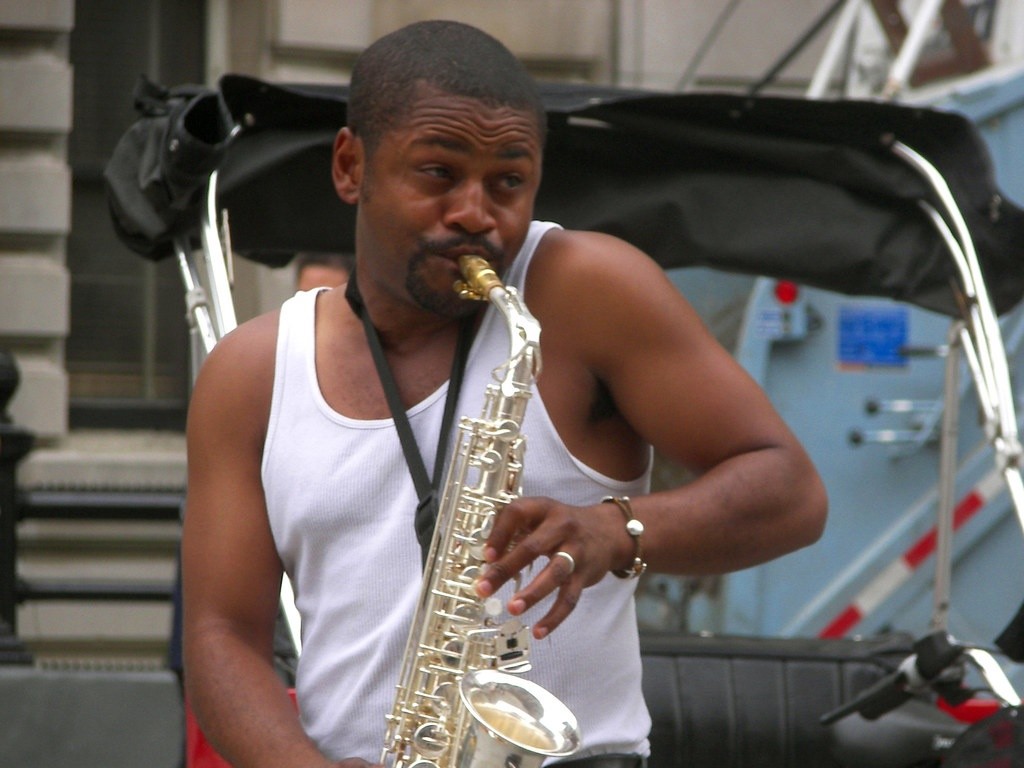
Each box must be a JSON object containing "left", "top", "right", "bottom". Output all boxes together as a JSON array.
[
  {"left": 181, "top": 20, "right": 828, "bottom": 768},
  {"left": 293, "top": 256, "right": 354, "bottom": 295}
]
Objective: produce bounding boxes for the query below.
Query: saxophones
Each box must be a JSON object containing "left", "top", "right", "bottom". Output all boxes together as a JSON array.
[{"left": 376, "top": 255, "right": 584, "bottom": 768}]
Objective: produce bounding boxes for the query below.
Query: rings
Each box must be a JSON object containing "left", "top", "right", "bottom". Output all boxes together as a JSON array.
[{"left": 555, "top": 551, "right": 576, "bottom": 577}]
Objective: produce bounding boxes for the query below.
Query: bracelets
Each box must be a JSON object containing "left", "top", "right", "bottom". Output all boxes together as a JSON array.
[{"left": 600, "top": 495, "right": 645, "bottom": 583}]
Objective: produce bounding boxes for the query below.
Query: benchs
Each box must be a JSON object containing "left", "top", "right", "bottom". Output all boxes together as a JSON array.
[{"left": 640, "top": 633, "right": 936, "bottom": 768}]
